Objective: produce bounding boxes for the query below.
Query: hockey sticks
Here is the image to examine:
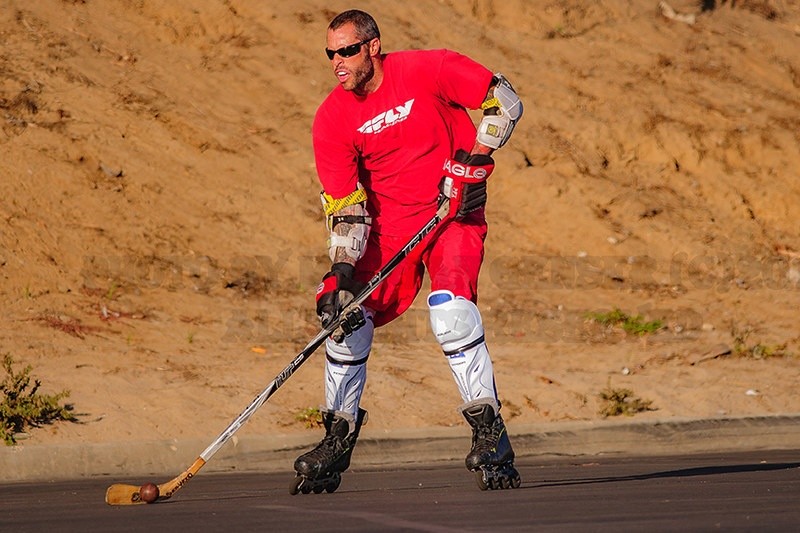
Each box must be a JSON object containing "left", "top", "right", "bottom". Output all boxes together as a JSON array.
[{"left": 106, "top": 202, "right": 451, "bottom": 507}]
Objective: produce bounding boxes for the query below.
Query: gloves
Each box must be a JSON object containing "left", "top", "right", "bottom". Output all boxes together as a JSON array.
[
  {"left": 313, "top": 271, "right": 368, "bottom": 343},
  {"left": 433, "top": 148, "right": 497, "bottom": 218}
]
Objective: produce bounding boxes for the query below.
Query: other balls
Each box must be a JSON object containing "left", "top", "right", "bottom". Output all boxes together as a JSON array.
[{"left": 140, "top": 483, "right": 159, "bottom": 504}]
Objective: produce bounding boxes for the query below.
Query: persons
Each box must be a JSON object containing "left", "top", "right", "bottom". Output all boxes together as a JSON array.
[{"left": 290, "top": 10, "right": 524, "bottom": 495}]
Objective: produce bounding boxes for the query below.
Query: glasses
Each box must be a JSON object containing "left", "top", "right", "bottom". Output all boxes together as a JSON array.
[{"left": 325, "top": 39, "right": 374, "bottom": 60}]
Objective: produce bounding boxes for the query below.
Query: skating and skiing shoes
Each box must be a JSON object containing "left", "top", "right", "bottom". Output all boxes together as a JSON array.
[
  {"left": 289, "top": 407, "right": 370, "bottom": 496},
  {"left": 459, "top": 395, "right": 522, "bottom": 490}
]
